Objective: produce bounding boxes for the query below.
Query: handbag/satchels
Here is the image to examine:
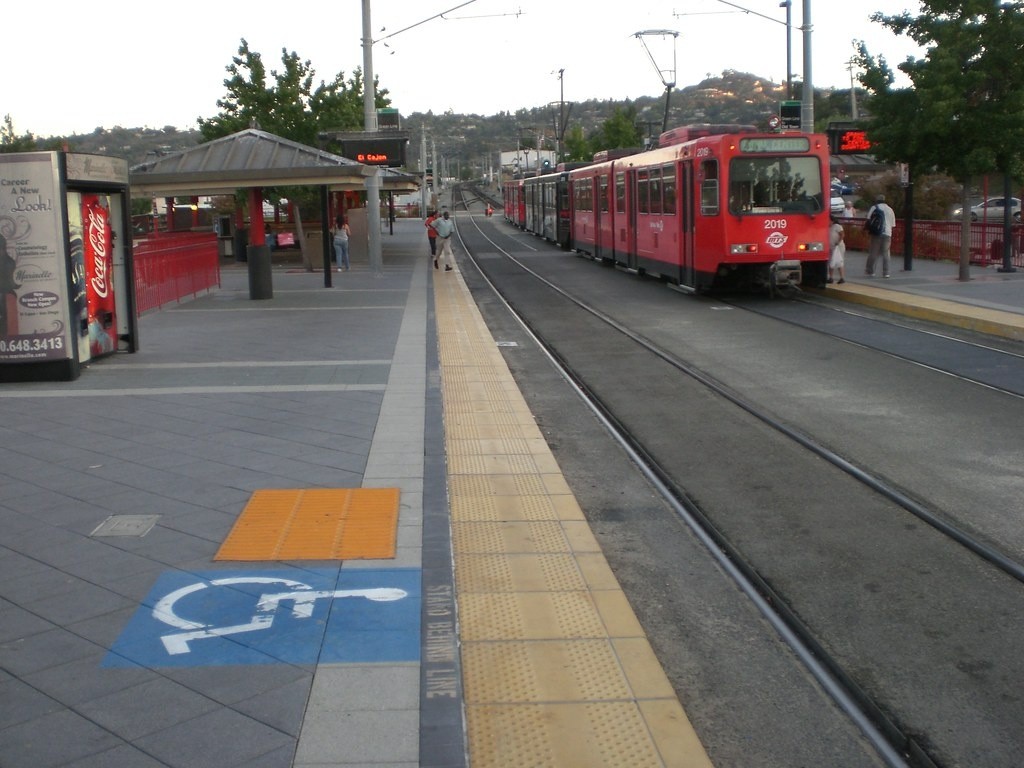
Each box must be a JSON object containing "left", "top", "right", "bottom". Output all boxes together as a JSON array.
[{"left": 830, "top": 245, "right": 843, "bottom": 269}]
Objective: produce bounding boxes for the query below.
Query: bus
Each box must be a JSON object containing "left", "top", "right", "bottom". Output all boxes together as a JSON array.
[
  {"left": 572, "top": 28, "right": 832, "bottom": 299},
  {"left": 523, "top": 68, "right": 595, "bottom": 248},
  {"left": 502, "top": 179, "right": 529, "bottom": 229}
]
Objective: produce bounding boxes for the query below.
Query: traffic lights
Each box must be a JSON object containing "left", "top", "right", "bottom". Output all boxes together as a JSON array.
[
  {"left": 489, "top": 209, "right": 492, "bottom": 216},
  {"left": 544, "top": 160, "right": 549, "bottom": 166}
]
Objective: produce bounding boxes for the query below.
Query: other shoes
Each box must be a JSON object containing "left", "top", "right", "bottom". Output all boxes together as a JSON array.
[
  {"left": 346, "top": 267, "right": 351, "bottom": 271},
  {"left": 826, "top": 278, "right": 833, "bottom": 283},
  {"left": 338, "top": 269, "right": 343, "bottom": 273},
  {"left": 434, "top": 260, "right": 438, "bottom": 269},
  {"left": 837, "top": 279, "right": 845, "bottom": 284},
  {"left": 431, "top": 254, "right": 435, "bottom": 258},
  {"left": 864, "top": 271, "right": 875, "bottom": 276},
  {"left": 880, "top": 272, "right": 890, "bottom": 278},
  {"left": 445, "top": 267, "right": 453, "bottom": 271}
]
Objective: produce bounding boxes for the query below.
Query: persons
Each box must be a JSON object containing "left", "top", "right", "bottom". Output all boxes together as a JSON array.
[
  {"left": 429, "top": 212, "right": 455, "bottom": 271},
  {"left": 842, "top": 201, "right": 857, "bottom": 217},
  {"left": 425, "top": 210, "right": 439, "bottom": 257},
  {"left": 332, "top": 214, "right": 351, "bottom": 272},
  {"left": 827, "top": 215, "right": 846, "bottom": 283},
  {"left": 866, "top": 195, "right": 896, "bottom": 278},
  {"left": 1010, "top": 216, "right": 1020, "bottom": 258}
]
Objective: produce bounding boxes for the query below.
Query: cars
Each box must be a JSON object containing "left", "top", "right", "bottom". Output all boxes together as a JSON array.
[
  {"left": 831, "top": 182, "right": 854, "bottom": 195},
  {"left": 830, "top": 188, "right": 846, "bottom": 213},
  {"left": 954, "top": 196, "right": 1023, "bottom": 223},
  {"left": 832, "top": 177, "right": 841, "bottom": 184}
]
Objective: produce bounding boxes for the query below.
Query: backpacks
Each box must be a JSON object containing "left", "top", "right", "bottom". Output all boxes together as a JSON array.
[{"left": 868, "top": 205, "right": 885, "bottom": 236}]
